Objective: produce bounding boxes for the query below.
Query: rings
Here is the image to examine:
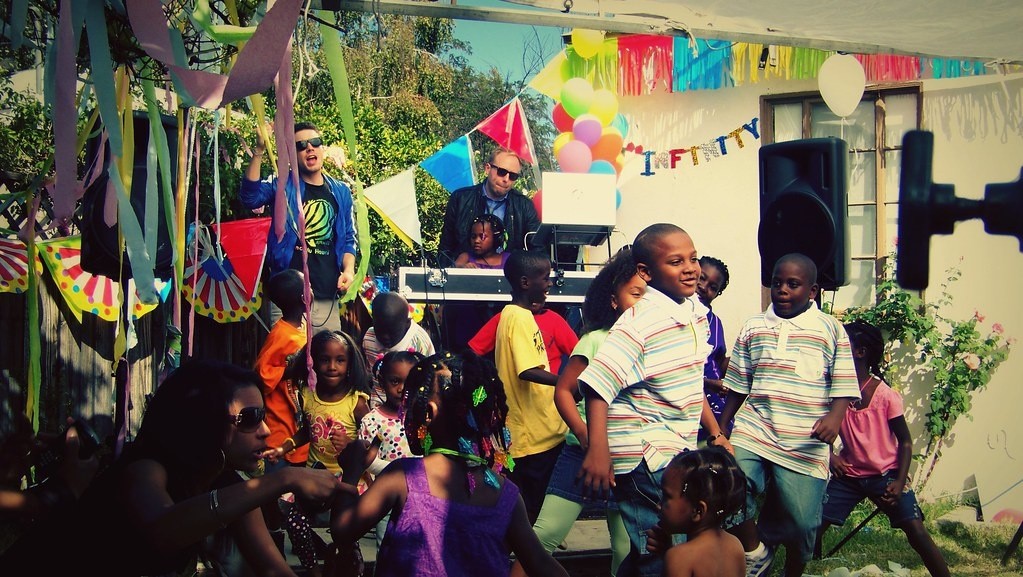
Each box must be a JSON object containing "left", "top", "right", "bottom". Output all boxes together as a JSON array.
[{"left": 321, "top": 502, "right": 326, "bottom": 509}]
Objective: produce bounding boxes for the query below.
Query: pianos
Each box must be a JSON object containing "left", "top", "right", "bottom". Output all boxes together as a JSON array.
[{"left": 388, "top": 264, "right": 604, "bottom": 306}]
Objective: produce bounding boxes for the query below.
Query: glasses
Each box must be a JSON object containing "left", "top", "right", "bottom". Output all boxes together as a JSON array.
[
  {"left": 491, "top": 164, "right": 519, "bottom": 182},
  {"left": 296, "top": 138, "right": 322, "bottom": 153},
  {"left": 219, "top": 406, "right": 271, "bottom": 434}
]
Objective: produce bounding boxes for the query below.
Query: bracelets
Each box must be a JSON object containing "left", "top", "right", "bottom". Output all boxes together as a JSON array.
[
  {"left": 252, "top": 148, "right": 266, "bottom": 156},
  {"left": 208, "top": 489, "right": 224, "bottom": 529},
  {"left": 283, "top": 437, "right": 296, "bottom": 455},
  {"left": 707, "top": 431, "right": 724, "bottom": 443},
  {"left": 211, "top": 490, "right": 227, "bottom": 529}
]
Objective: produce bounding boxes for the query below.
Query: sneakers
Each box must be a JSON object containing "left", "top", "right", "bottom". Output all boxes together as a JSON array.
[{"left": 744, "top": 545, "right": 775, "bottom": 577}]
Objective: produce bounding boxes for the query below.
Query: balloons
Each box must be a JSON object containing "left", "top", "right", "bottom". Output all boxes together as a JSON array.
[
  {"left": 552, "top": 29, "right": 628, "bottom": 209},
  {"left": 818, "top": 54, "right": 865, "bottom": 117}
]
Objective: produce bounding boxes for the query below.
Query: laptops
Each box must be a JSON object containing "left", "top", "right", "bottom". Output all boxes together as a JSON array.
[{"left": 531, "top": 172, "right": 616, "bottom": 247}]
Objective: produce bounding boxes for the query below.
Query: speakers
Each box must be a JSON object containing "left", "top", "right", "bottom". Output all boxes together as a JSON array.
[
  {"left": 756, "top": 135, "right": 852, "bottom": 291},
  {"left": 81, "top": 108, "right": 186, "bottom": 282}
]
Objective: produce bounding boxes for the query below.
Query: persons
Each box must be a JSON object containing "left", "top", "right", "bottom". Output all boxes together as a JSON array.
[{"left": 0, "top": 124, "right": 952, "bottom": 577}]
